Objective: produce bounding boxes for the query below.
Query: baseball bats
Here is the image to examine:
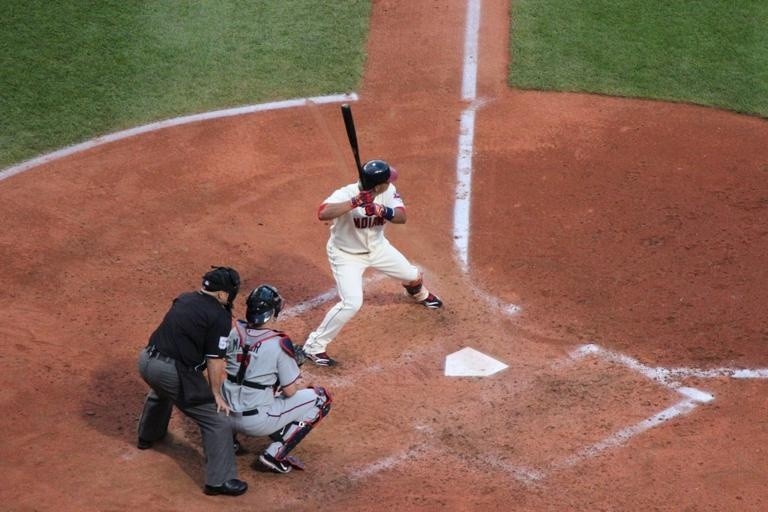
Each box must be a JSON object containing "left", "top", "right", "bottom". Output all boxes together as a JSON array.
[{"left": 341, "top": 104, "right": 374, "bottom": 215}]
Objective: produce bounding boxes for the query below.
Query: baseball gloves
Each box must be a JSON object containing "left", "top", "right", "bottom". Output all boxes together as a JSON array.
[{"left": 292, "top": 344, "right": 306, "bottom": 366}]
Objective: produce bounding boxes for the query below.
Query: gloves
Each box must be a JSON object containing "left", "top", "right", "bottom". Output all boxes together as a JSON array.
[
  {"left": 365, "top": 203, "right": 386, "bottom": 218},
  {"left": 351, "top": 191, "right": 374, "bottom": 208}
]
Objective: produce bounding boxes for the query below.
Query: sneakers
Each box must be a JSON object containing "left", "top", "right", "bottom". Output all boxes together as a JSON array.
[
  {"left": 305, "top": 353, "right": 335, "bottom": 366},
  {"left": 405, "top": 290, "right": 442, "bottom": 309},
  {"left": 233, "top": 441, "right": 240, "bottom": 452},
  {"left": 139, "top": 433, "right": 163, "bottom": 451},
  {"left": 204, "top": 479, "right": 248, "bottom": 496},
  {"left": 258, "top": 452, "right": 292, "bottom": 473}
]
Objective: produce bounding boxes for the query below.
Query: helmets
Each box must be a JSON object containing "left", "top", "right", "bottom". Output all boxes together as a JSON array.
[
  {"left": 247, "top": 284, "right": 286, "bottom": 326},
  {"left": 201, "top": 266, "right": 240, "bottom": 309},
  {"left": 359, "top": 160, "right": 398, "bottom": 191}
]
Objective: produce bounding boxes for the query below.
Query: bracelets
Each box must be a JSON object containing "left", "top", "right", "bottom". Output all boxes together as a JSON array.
[{"left": 383, "top": 206, "right": 395, "bottom": 222}]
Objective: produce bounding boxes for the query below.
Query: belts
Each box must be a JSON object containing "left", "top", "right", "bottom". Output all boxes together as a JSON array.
[
  {"left": 144, "top": 345, "right": 171, "bottom": 363},
  {"left": 242, "top": 408, "right": 259, "bottom": 416}
]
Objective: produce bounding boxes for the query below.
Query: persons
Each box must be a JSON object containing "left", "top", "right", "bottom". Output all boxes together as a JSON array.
[
  {"left": 135, "top": 265, "right": 248, "bottom": 496},
  {"left": 294, "top": 158, "right": 448, "bottom": 367},
  {"left": 215, "top": 283, "right": 332, "bottom": 474}
]
